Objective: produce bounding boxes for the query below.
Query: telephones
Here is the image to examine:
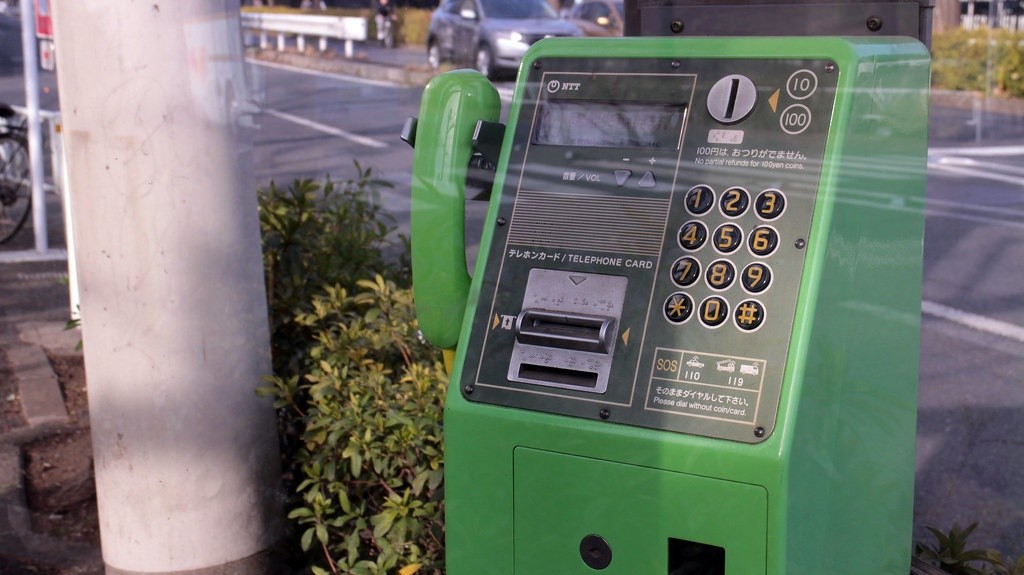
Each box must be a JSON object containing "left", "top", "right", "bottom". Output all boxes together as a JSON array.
[{"left": 399, "top": 34, "right": 935, "bottom": 575}]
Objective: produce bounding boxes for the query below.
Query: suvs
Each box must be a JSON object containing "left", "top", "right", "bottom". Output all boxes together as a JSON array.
[{"left": 426, "top": 0, "right": 585, "bottom": 80}]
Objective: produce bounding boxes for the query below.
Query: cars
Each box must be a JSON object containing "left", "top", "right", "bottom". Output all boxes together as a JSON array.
[{"left": 563, "top": 0, "right": 628, "bottom": 37}]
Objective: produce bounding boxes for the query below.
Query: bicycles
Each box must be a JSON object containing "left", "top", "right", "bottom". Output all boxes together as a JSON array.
[
  {"left": 377, "top": 19, "right": 397, "bottom": 51},
  {"left": 0, "top": 100, "right": 32, "bottom": 248}
]
